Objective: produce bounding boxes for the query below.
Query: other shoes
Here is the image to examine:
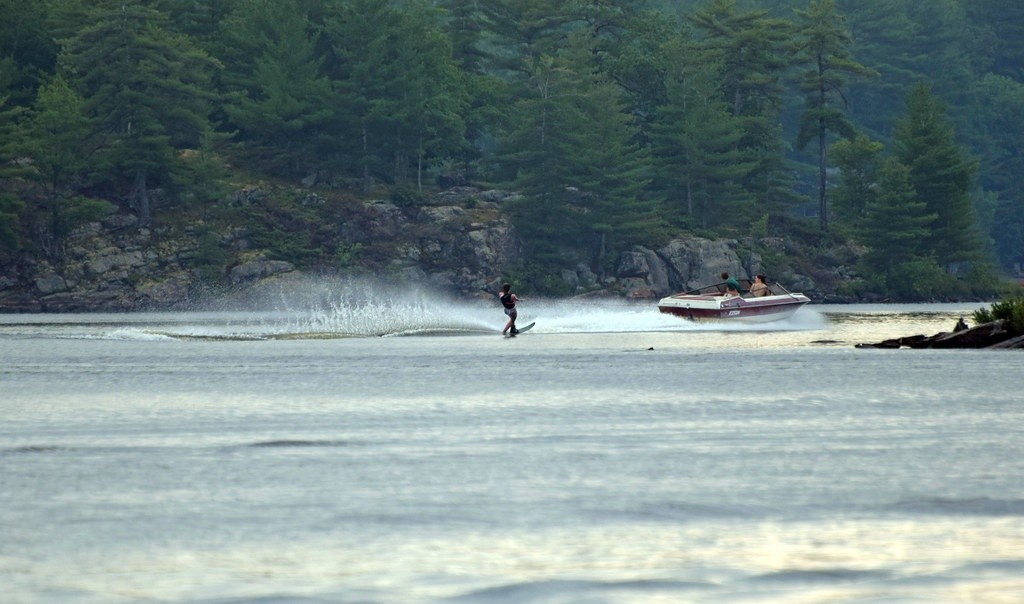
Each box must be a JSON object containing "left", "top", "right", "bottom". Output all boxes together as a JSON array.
[{"left": 510, "top": 326, "right": 519, "bottom": 333}]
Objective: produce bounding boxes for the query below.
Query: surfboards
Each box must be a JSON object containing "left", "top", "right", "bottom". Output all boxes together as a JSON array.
[{"left": 503, "top": 322, "right": 536, "bottom": 340}]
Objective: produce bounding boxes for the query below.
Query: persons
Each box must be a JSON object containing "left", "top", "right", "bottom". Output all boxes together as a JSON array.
[
  {"left": 499, "top": 283, "right": 520, "bottom": 335},
  {"left": 750, "top": 274, "right": 771, "bottom": 296},
  {"left": 724, "top": 285, "right": 739, "bottom": 298},
  {"left": 722, "top": 272, "right": 744, "bottom": 298}
]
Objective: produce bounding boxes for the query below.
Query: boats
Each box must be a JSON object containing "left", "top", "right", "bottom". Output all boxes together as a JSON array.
[{"left": 658, "top": 276, "right": 813, "bottom": 328}]
